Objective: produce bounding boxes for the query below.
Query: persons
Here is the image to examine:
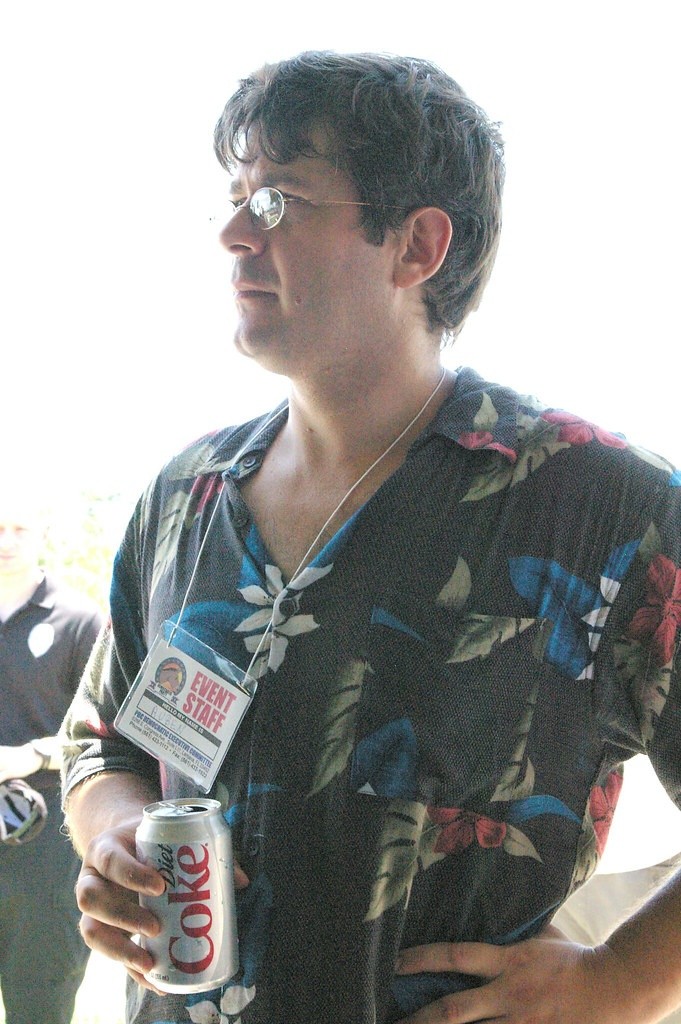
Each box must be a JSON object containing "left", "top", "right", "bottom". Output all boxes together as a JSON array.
[
  {"left": 48, "top": 49, "right": 680, "bottom": 1024},
  {"left": 0, "top": 481, "right": 109, "bottom": 1024}
]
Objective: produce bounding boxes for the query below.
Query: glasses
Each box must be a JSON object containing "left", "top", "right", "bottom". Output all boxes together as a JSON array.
[{"left": 210, "top": 187, "right": 411, "bottom": 231}]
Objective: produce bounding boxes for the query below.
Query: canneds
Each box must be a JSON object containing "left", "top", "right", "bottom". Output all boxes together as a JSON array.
[{"left": 134, "top": 797, "right": 239, "bottom": 994}]
